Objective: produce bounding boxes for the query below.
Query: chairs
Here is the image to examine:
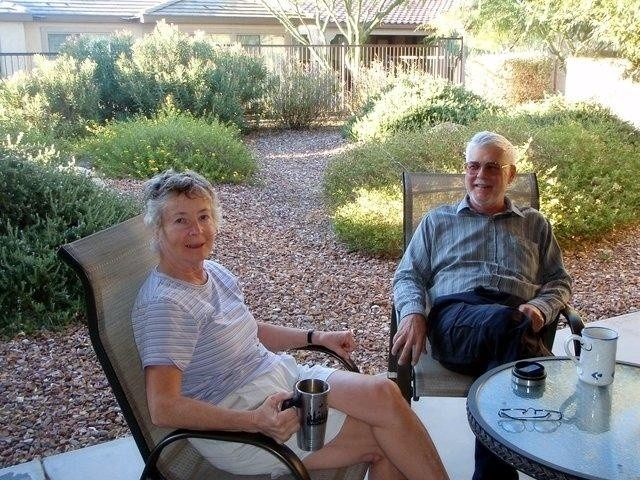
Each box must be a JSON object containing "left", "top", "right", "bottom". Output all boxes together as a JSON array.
[
  {"left": 57, "top": 210, "right": 371, "bottom": 480},
  {"left": 387, "top": 171, "right": 584, "bottom": 408}
]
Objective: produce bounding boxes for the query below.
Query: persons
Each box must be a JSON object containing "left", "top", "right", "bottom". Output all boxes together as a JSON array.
[
  {"left": 385, "top": 131, "right": 573, "bottom": 478},
  {"left": 130, "top": 165, "right": 454, "bottom": 480}
]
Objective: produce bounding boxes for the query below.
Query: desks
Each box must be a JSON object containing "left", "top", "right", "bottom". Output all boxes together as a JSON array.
[{"left": 466, "top": 356, "right": 640, "bottom": 480}]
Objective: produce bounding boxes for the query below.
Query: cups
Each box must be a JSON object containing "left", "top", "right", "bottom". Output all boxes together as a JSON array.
[
  {"left": 565, "top": 326, "right": 619, "bottom": 387},
  {"left": 281, "top": 377, "right": 331, "bottom": 450}
]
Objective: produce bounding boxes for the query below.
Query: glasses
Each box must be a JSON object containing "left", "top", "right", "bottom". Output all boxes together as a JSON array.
[{"left": 463, "top": 162, "right": 512, "bottom": 176}]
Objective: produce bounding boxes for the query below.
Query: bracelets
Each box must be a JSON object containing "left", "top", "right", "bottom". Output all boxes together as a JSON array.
[{"left": 306, "top": 329, "right": 314, "bottom": 345}]
